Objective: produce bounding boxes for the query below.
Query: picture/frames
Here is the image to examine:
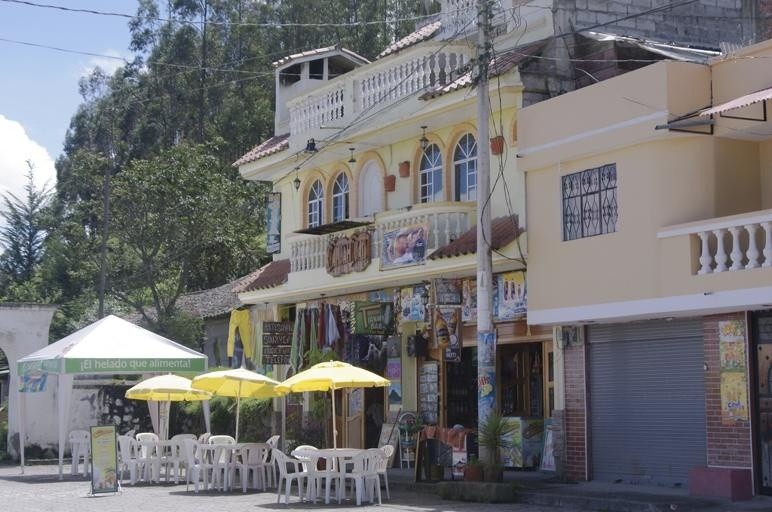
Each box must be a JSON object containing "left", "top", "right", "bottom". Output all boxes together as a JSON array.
[{"left": 378, "top": 221, "right": 429, "bottom": 270}]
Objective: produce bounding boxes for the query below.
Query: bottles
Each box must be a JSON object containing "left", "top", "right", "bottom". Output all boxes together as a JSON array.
[
  {"left": 384, "top": 303, "right": 392, "bottom": 325},
  {"left": 435, "top": 310, "right": 451, "bottom": 347},
  {"left": 448, "top": 308, "right": 457, "bottom": 335},
  {"left": 386, "top": 229, "right": 422, "bottom": 262}
]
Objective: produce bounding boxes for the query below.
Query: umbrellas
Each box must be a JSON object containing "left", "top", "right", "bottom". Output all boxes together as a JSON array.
[
  {"left": 124, "top": 372, "right": 212, "bottom": 455},
  {"left": 274, "top": 359, "right": 392, "bottom": 450},
  {"left": 191, "top": 366, "right": 282, "bottom": 446}
]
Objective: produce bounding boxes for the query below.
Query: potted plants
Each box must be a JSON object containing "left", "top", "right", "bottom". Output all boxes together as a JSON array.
[{"left": 460, "top": 413, "right": 509, "bottom": 480}]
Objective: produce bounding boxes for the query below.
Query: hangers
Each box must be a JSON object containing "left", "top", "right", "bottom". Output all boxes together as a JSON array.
[{"left": 231, "top": 303, "right": 247, "bottom": 312}]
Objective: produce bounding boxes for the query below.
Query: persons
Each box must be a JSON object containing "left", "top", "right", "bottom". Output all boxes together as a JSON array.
[
  {"left": 385, "top": 227, "right": 426, "bottom": 266},
  {"left": 502, "top": 281, "right": 522, "bottom": 301}
]
